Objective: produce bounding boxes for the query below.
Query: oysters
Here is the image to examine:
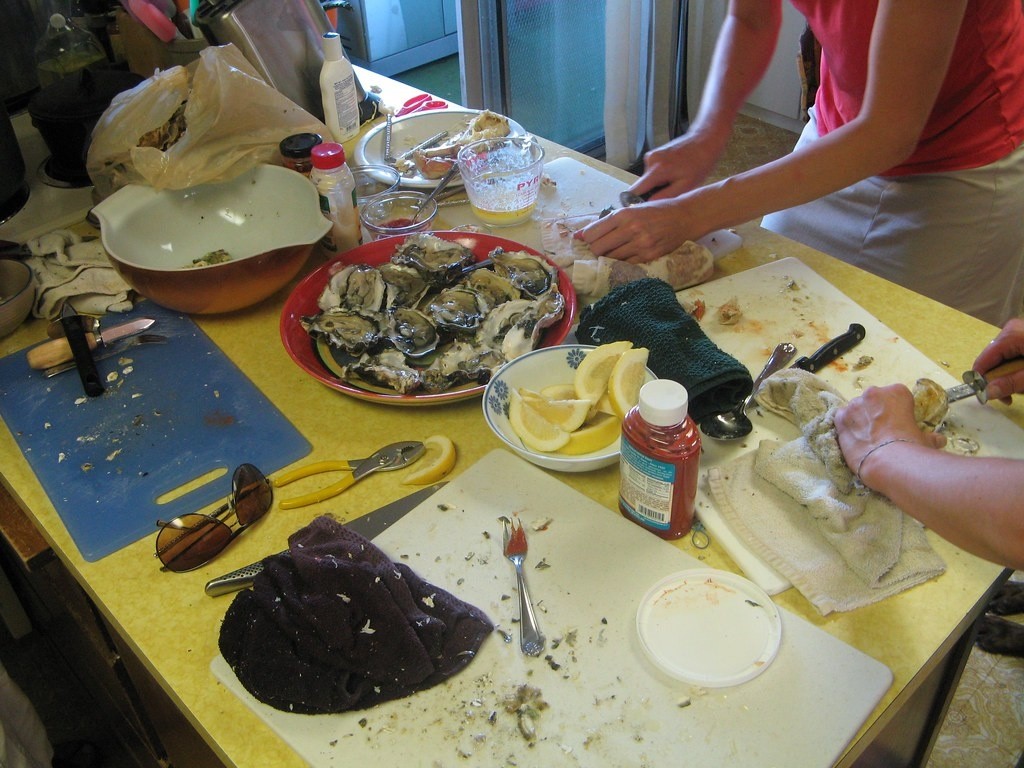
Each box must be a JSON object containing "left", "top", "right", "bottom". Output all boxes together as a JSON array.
[
  {"left": 86, "top": 98, "right": 188, "bottom": 187},
  {"left": 912, "top": 378, "right": 949, "bottom": 433},
  {"left": 299, "top": 232, "right": 565, "bottom": 395}
]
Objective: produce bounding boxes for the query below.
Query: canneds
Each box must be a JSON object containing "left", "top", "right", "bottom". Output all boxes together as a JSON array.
[{"left": 278, "top": 132, "right": 322, "bottom": 180}]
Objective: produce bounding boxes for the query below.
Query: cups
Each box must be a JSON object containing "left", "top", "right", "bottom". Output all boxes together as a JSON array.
[
  {"left": 457, "top": 137, "right": 544, "bottom": 228},
  {"left": 349, "top": 164, "right": 401, "bottom": 212},
  {"left": 360, "top": 191, "right": 437, "bottom": 243}
]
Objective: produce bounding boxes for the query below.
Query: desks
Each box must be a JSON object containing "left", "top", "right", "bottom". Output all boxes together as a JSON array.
[{"left": 0, "top": 62, "right": 1024, "bottom": 768}]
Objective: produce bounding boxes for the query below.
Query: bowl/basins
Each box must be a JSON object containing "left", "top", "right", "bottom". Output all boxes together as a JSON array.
[
  {"left": 483, "top": 344, "right": 659, "bottom": 473},
  {"left": 92, "top": 164, "right": 333, "bottom": 315},
  {"left": 0, "top": 259, "right": 35, "bottom": 338},
  {"left": 29, "top": 69, "right": 148, "bottom": 180}
]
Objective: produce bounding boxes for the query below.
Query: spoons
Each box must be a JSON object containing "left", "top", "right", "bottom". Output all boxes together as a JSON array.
[{"left": 699, "top": 342, "right": 796, "bottom": 441}]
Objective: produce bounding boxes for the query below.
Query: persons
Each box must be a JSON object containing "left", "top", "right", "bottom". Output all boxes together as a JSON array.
[
  {"left": 832, "top": 314, "right": 1024, "bottom": 572},
  {"left": 573, "top": 0, "right": 1024, "bottom": 270}
]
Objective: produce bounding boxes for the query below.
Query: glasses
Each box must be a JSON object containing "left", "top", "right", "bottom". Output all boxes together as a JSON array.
[{"left": 153, "top": 464, "right": 273, "bottom": 573}]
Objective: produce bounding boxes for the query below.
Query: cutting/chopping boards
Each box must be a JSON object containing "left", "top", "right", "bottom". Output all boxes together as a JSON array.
[
  {"left": 0, "top": 300, "right": 312, "bottom": 563},
  {"left": 209, "top": 449, "right": 891, "bottom": 768},
  {"left": 675, "top": 258, "right": 1023, "bottom": 599},
  {"left": 439, "top": 157, "right": 741, "bottom": 284}
]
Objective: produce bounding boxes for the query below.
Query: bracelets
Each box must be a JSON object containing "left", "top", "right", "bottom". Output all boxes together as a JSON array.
[{"left": 854, "top": 438, "right": 917, "bottom": 495}]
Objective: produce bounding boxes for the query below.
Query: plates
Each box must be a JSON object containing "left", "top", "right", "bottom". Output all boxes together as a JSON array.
[
  {"left": 354, "top": 108, "right": 526, "bottom": 188},
  {"left": 278, "top": 232, "right": 575, "bottom": 407}
]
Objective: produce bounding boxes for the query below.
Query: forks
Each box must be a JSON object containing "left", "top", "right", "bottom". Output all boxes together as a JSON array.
[
  {"left": 503, "top": 518, "right": 544, "bottom": 656},
  {"left": 43, "top": 334, "right": 167, "bottom": 378}
]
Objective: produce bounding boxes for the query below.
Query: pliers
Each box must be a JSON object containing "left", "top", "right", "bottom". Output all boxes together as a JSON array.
[{"left": 271, "top": 436, "right": 433, "bottom": 508}]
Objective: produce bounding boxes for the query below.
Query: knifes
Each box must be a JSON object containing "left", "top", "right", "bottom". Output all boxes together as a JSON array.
[
  {"left": 745, "top": 323, "right": 866, "bottom": 410},
  {"left": 28, "top": 318, "right": 155, "bottom": 370},
  {"left": 938, "top": 356, "right": 1024, "bottom": 403},
  {"left": 203, "top": 481, "right": 449, "bottom": 598}
]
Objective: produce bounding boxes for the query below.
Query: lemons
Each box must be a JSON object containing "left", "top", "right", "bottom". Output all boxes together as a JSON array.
[{"left": 509, "top": 340, "right": 648, "bottom": 455}]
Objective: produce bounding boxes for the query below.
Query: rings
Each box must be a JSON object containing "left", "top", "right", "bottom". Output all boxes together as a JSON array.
[{"left": 990, "top": 340, "right": 994, "bottom": 344}]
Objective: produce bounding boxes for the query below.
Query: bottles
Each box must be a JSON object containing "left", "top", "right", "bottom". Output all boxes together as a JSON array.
[
  {"left": 106, "top": 6, "right": 128, "bottom": 70},
  {"left": 320, "top": 31, "right": 360, "bottom": 144},
  {"left": 27, "top": 0, "right": 112, "bottom": 112},
  {"left": 310, "top": 143, "right": 364, "bottom": 265}
]
